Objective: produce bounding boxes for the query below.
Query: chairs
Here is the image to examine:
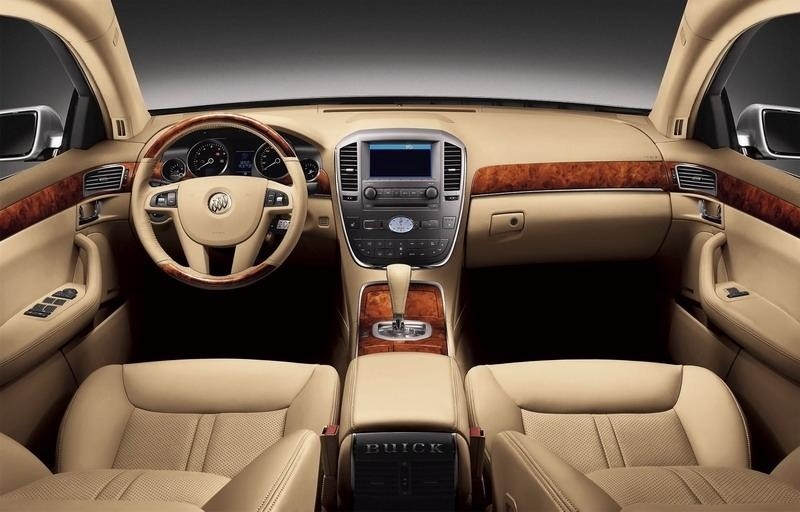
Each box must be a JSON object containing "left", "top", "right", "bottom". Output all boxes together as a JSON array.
[
  {"left": 463, "top": 359, "right": 800, "bottom": 512},
  {"left": 0, "top": 355, "right": 343, "bottom": 512}
]
